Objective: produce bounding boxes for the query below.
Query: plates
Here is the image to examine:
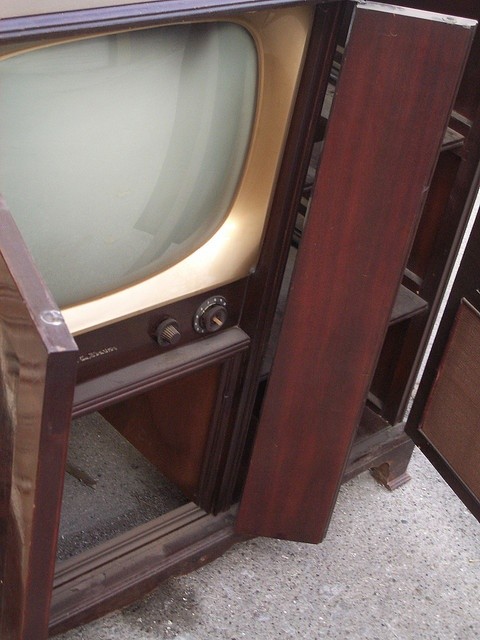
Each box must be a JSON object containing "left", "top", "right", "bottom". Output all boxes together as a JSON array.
[{"left": 1, "top": 2, "right": 479, "bottom": 639}]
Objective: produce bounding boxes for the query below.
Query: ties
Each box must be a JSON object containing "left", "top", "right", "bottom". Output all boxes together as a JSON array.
[{"left": 1, "top": 0, "right": 316, "bottom": 375}]
